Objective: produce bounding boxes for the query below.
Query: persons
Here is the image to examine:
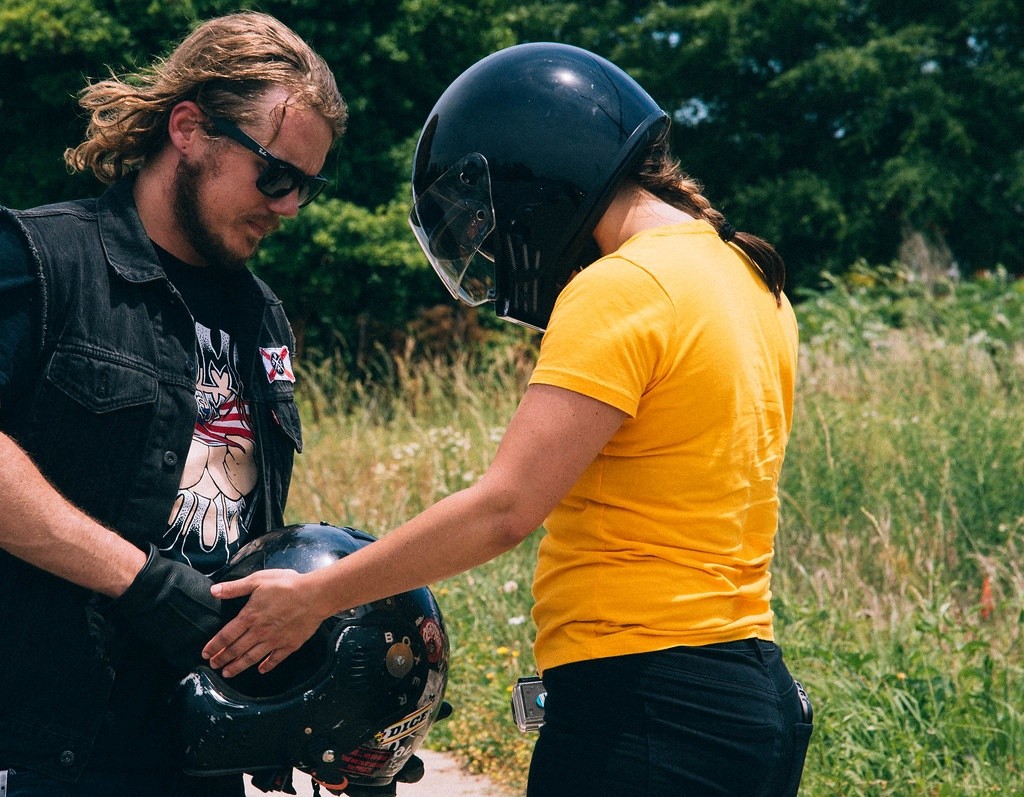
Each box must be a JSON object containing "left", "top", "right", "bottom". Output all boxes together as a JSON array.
[
  {"left": 203, "top": 39, "right": 813, "bottom": 796},
  {"left": 0, "top": 10, "right": 454, "bottom": 797}
]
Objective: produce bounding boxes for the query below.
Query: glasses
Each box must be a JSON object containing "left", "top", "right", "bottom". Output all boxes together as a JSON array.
[{"left": 208, "top": 114, "right": 329, "bottom": 210}]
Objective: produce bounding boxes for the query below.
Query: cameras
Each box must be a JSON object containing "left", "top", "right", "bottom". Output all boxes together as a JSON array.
[{"left": 512, "top": 680, "right": 549, "bottom": 732}]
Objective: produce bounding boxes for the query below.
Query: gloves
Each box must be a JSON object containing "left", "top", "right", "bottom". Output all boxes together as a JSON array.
[
  {"left": 347, "top": 701, "right": 453, "bottom": 797},
  {"left": 100, "top": 535, "right": 300, "bottom": 696}
]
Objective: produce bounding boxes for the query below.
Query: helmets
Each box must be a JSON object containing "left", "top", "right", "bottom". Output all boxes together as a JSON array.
[
  {"left": 419, "top": 41, "right": 671, "bottom": 336},
  {"left": 176, "top": 521, "right": 449, "bottom": 788}
]
